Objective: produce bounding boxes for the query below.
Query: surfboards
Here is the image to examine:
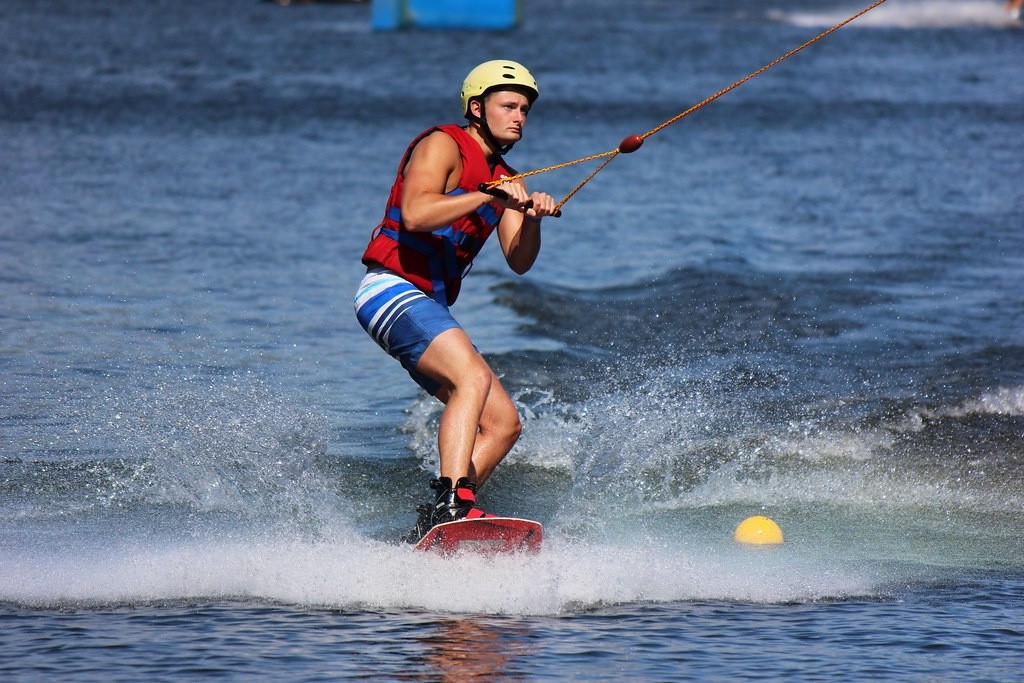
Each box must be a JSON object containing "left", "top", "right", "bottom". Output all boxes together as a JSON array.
[{"left": 412, "top": 517, "right": 543, "bottom": 559}]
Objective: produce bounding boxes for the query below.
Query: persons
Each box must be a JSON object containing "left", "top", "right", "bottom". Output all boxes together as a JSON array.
[{"left": 353, "top": 60, "right": 556, "bottom": 548}]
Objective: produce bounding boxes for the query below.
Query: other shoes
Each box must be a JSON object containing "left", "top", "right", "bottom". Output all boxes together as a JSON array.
[
  {"left": 431, "top": 475, "right": 499, "bottom": 524},
  {"left": 409, "top": 503, "right": 436, "bottom": 542}
]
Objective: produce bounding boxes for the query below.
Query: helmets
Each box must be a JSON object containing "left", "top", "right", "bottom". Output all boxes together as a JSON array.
[{"left": 460, "top": 60, "right": 539, "bottom": 119}]
